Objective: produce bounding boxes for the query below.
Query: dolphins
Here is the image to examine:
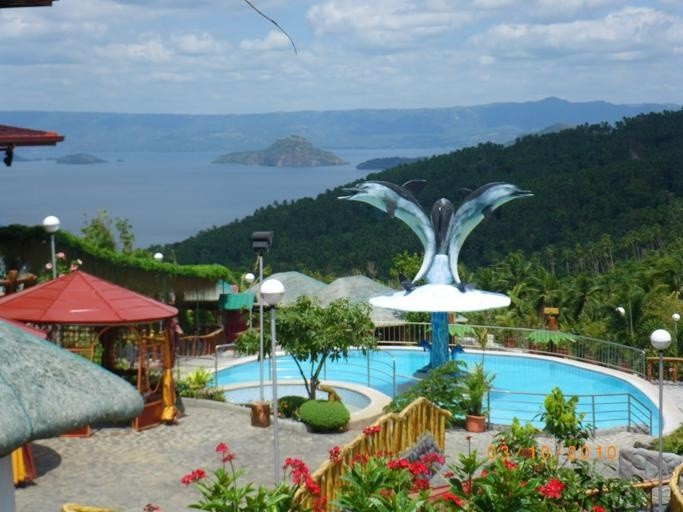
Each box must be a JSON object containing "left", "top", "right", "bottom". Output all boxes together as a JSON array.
[
  {"left": 448, "top": 181, "right": 536, "bottom": 293},
  {"left": 335, "top": 179, "right": 438, "bottom": 296},
  {"left": 430, "top": 197, "right": 455, "bottom": 255}
]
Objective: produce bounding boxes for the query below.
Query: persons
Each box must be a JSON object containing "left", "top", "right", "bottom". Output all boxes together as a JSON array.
[{"left": 173, "top": 318, "right": 184, "bottom": 359}]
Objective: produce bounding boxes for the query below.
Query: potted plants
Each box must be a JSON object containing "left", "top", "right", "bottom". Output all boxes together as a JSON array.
[
  {"left": 459, "top": 360, "right": 496, "bottom": 431},
  {"left": 500, "top": 325, "right": 633, "bottom": 372}
]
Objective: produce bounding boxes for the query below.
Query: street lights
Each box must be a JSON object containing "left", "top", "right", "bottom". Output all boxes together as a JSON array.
[
  {"left": 243, "top": 272, "right": 254, "bottom": 328},
  {"left": 673, "top": 312, "right": 682, "bottom": 357},
  {"left": 257, "top": 279, "right": 284, "bottom": 489},
  {"left": 614, "top": 306, "right": 627, "bottom": 318},
  {"left": 40, "top": 212, "right": 60, "bottom": 280},
  {"left": 251, "top": 226, "right": 273, "bottom": 402},
  {"left": 648, "top": 326, "right": 672, "bottom": 512}
]
